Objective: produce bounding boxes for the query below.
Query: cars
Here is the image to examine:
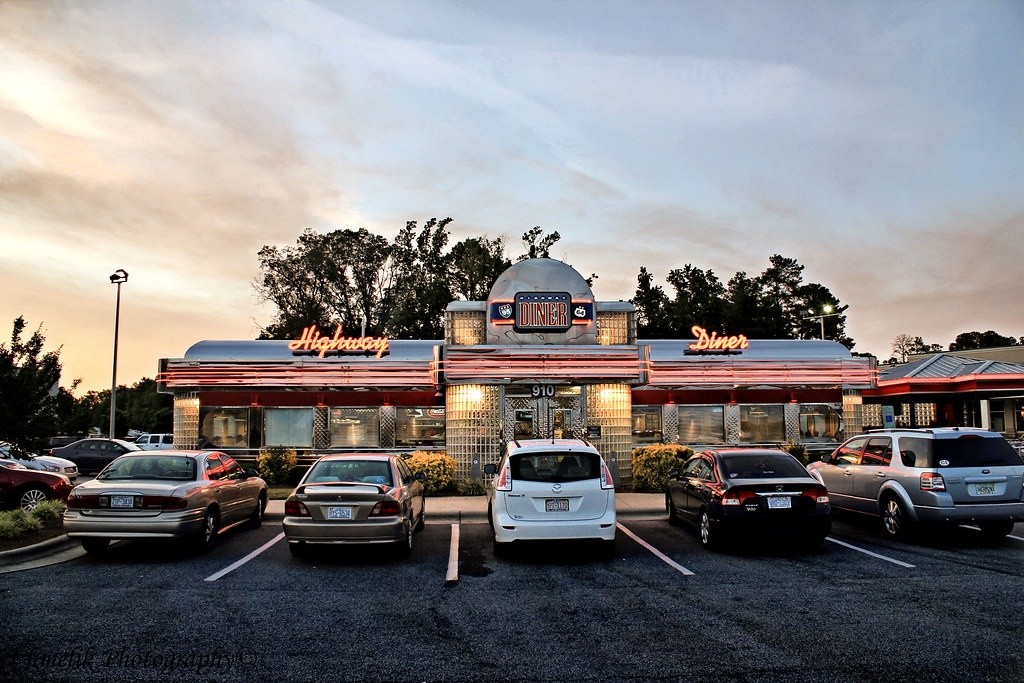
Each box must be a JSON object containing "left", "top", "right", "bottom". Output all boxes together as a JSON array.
[
  {"left": 664, "top": 447, "right": 832, "bottom": 551},
  {"left": 0, "top": 441, "right": 79, "bottom": 482},
  {"left": 282, "top": 453, "right": 428, "bottom": 559},
  {"left": 62, "top": 449, "right": 270, "bottom": 557},
  {"left": 0, "top": 456, "right": 75, "bottom": 514},
  {"left": 50, "top": 437, "right": 144, "bottom": 474}
]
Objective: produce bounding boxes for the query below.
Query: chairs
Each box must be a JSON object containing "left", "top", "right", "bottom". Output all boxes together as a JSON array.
[
  {"left": 562, "top": 465, "right": 587, "bottom": 481},
  {"left": 520, "top": 459, "right": 538, "bottom": 479},
  {"left": 557, "top": 456, "right": 578, "bottom": 475}
]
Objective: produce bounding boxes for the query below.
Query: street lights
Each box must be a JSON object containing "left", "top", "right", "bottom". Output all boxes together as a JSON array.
[
  {"left": 801, "top": 303, "right": 843, "bottom": 341},
  {"left": 108, "top": 268, "right": 130, "bottom": 438}
]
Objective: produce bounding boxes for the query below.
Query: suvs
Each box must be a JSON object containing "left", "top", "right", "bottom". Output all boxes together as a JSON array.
[
  {"left": 113, "top": 432, "right": 173, "bottom": 451},
  {"left": 484, "top": 437, "right": 616, "bottom": 559},
  {"left": 805, "top": 425, "right": 1024, "bottom": 541}
]
